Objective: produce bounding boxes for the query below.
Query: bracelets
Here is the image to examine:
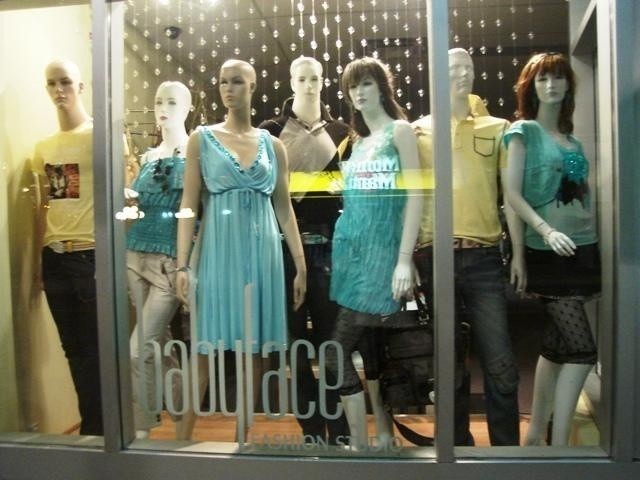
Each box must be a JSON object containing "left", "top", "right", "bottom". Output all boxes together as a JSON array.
[{"left": 543, "top": 227, "right": 557, "bottom": 247}]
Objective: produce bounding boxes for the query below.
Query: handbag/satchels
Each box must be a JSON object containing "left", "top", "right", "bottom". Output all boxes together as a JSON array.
[{"left": 378, "top": 298, "right": 468, "bottom": 406}]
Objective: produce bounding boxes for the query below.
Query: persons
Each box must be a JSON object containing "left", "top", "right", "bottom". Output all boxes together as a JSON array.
[
  {"left": 505, "top": 53, "right": 603, "bottom": 446},
  {"left": 30, "top": 60, "right": 131, "bottom": 436},
  {"left": 411, "top": 49, "right": 524, "bottom": 446},
  {"left": 138, "top": 59, "right": 423, "bottom": 444}
]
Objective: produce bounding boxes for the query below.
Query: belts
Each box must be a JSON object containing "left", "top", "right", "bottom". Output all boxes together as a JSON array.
[{"left": 452, "top": 238, "right": 479, "bottom": 250}]
